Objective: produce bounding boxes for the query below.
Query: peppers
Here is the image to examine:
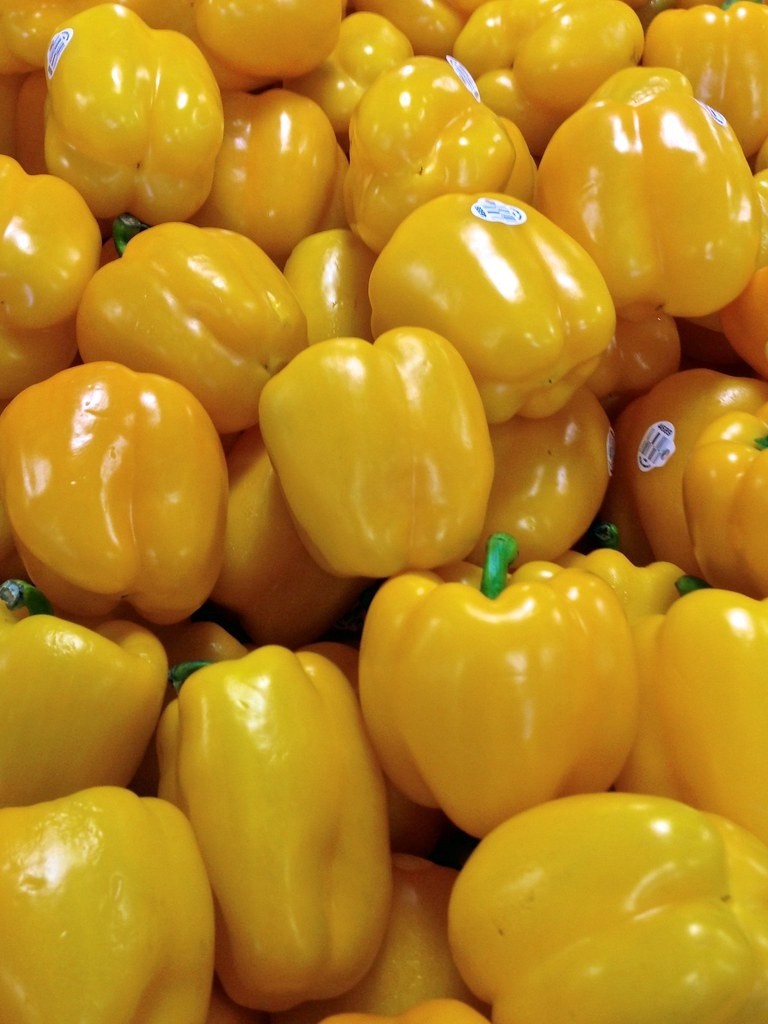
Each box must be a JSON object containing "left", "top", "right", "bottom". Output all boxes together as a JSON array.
[{"left": 0, "top": 0, "right": 768, "bottom": 1024}]
